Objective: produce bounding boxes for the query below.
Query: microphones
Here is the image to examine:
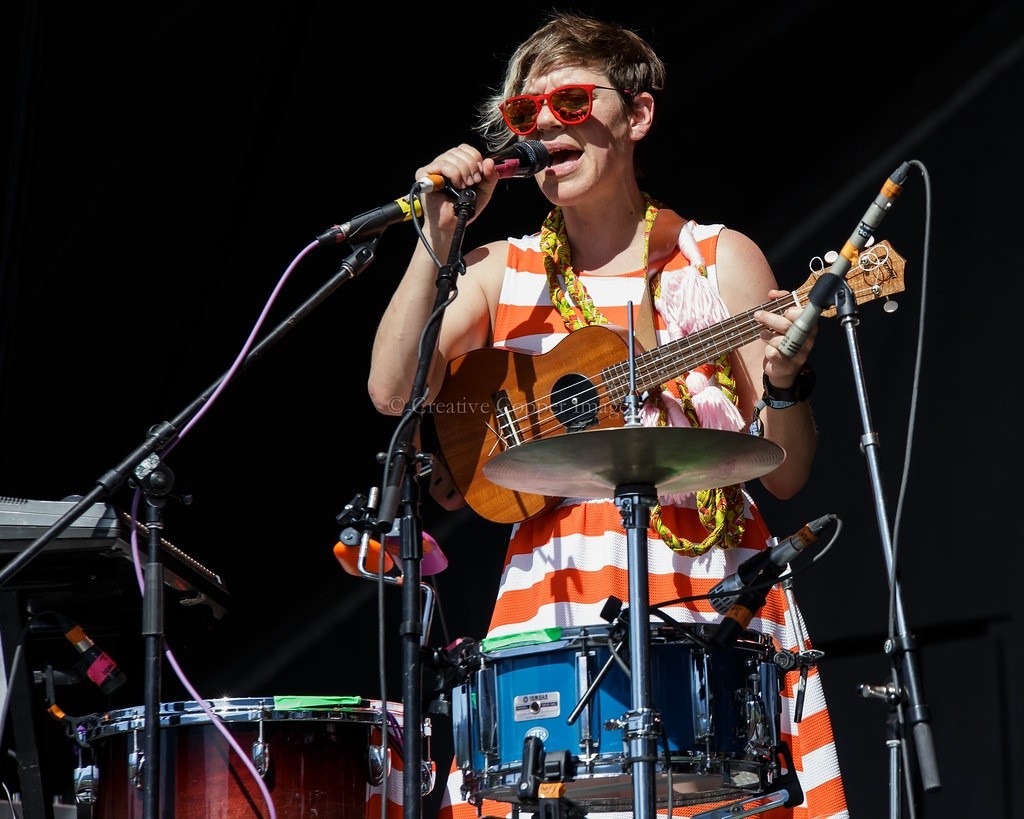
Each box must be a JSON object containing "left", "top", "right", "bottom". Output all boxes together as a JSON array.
[
  {"left": 316, "top": 190, "right": 422, "bottom": 246},
  {"left": 54, "top": 614, "right": 127, "bottom": 694},
  {"left": 777, "top": 160, "right": 911, "bottom": 358},
  {"left": 415, "top": 141, "right": 551, "bottom": 193},
  {"left": 707, "top": 513, "right": 840, "bottom": 615}
]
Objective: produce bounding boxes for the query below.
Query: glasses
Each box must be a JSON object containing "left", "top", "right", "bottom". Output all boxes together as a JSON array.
[{"left": 498, "top": 85, "right": 635, "bottom": 136}]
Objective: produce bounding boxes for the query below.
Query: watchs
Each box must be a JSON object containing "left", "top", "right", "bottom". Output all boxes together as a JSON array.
[{"left": 759, "top": 362, "right": 816, "bottom": 408}]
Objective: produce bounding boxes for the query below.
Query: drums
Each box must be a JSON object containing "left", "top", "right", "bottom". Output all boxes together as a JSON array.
[
  {"left": 448, "top": 622, "right": 780, "bottom": 812},
  {"left": 72, "top": 695, "right": 431, "bottom": 819}
]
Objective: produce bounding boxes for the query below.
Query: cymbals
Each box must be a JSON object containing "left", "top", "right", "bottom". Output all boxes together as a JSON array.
[{"left": 480, "top": 423, "right": 787, "bottom": 499}]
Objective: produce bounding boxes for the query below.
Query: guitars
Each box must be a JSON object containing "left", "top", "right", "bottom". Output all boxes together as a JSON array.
[{"left": 430, "top": 238, "right": 905, "bottom": 524}]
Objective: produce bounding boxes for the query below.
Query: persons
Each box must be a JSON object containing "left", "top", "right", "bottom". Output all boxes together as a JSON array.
[{"left": 370, "top": 19, "right": 849, "bottom": 819}]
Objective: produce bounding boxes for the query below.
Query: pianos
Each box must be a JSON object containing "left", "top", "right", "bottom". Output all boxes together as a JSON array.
[{"left": 0, "top": 494, "right": 234, "bottom": 624}]
